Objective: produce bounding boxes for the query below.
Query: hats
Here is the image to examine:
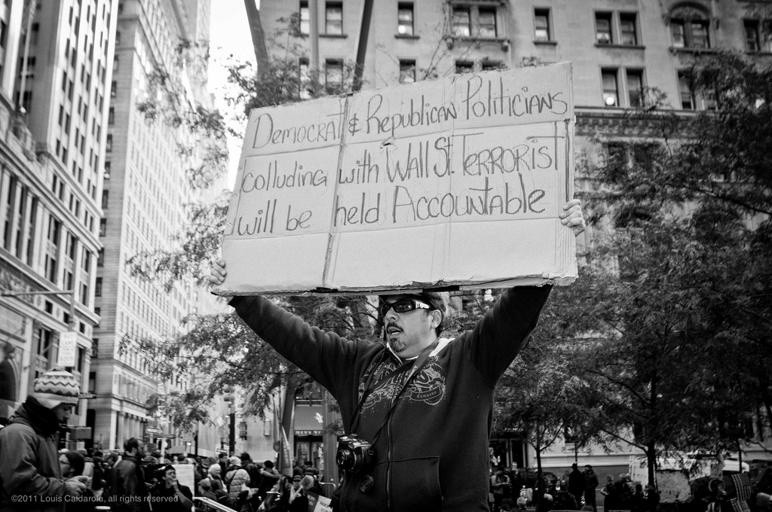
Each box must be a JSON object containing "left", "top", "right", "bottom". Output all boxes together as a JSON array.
[{"left": 31, "top": 364, "right": 79, "bottom": 409}]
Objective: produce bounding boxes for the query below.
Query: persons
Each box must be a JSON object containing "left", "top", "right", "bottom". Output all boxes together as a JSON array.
[
  {"left": 1, "top": 366, "right": 771, "bottom": 512},
  {"left": 208, "top": 196, "right": 587, "bottom": 511}
]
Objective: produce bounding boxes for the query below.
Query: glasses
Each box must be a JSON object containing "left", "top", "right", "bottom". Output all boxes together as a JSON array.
[{"left": 380, "top": 298, "right": 434, "bottom": 317}]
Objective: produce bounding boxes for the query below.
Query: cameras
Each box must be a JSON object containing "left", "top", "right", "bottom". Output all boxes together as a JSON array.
[{"left": 334, "top": 434, "right": 374, "bottom": 478}]
